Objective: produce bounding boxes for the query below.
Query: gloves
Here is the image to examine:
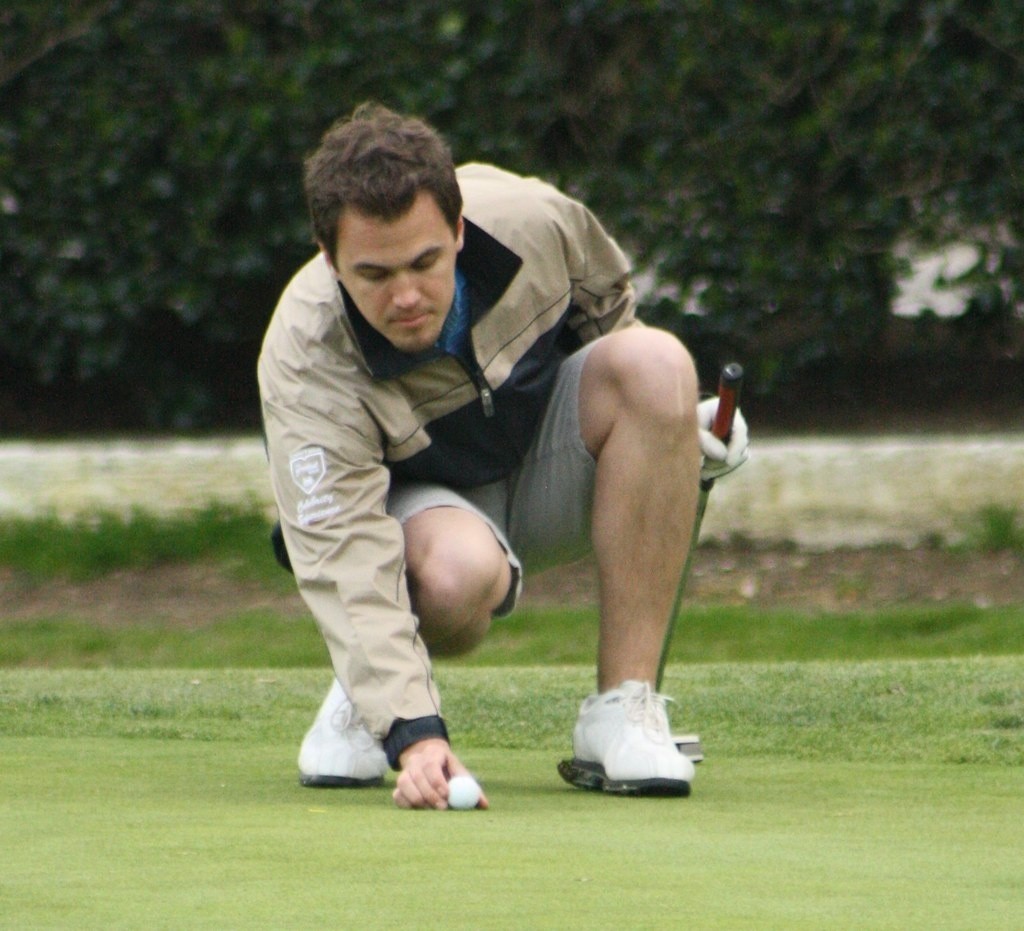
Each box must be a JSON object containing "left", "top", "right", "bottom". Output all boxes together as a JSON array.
[{"left": 695, "top": 397, "right": 748, "bottom": 482}]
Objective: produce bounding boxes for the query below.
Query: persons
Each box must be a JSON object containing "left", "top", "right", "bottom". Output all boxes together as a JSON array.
[{"left": 257, "top": 99, "right": 749, "bottom": 812}]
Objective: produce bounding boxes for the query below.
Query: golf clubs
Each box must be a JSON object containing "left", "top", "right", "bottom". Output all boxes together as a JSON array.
[{"left": 656, "top": 365, "right": 744, "bottom": 763}]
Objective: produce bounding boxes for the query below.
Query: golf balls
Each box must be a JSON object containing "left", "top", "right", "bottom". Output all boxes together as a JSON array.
[{"left": 448, "top": 775, "right": 480, "bottom": 810}]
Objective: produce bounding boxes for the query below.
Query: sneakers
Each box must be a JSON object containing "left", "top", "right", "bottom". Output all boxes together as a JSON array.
[
  {"left": 557, "top": 680, "right": 694, "bottom": 797},
  {"left": 298, "top": 679, "right": 387, "bottom": 787}
]
